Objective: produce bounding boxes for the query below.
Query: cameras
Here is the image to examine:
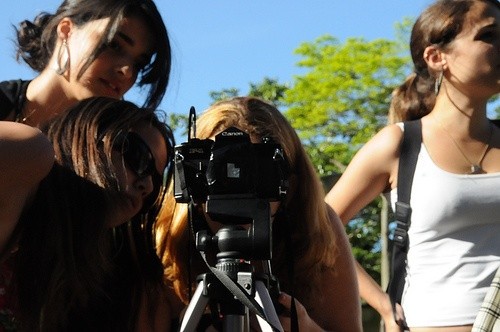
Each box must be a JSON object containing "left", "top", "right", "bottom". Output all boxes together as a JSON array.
[{"left": 171, "top": 130, "right": 287, "bottom": 257}]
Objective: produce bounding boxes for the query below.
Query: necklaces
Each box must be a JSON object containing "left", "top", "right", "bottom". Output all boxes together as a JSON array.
[{"left": 431, "top": 111, "right": 494, "bottom": 174}]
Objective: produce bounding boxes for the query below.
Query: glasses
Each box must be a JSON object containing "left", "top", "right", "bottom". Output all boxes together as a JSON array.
[{"left": 111, "top": 132, "right": 164, "bottom": 216}]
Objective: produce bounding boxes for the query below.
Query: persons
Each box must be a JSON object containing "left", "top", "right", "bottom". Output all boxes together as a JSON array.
[
  {"left": 0, "top": 0, "right": 364, "bottom": 332},
  {"left": 325, "top": 0, "right": 500, "bottom": 332}
]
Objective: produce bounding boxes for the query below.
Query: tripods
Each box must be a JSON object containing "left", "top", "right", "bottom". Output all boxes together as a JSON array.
[{"left": 179, "top": 257, "right": 285, "bottom": 332}]
